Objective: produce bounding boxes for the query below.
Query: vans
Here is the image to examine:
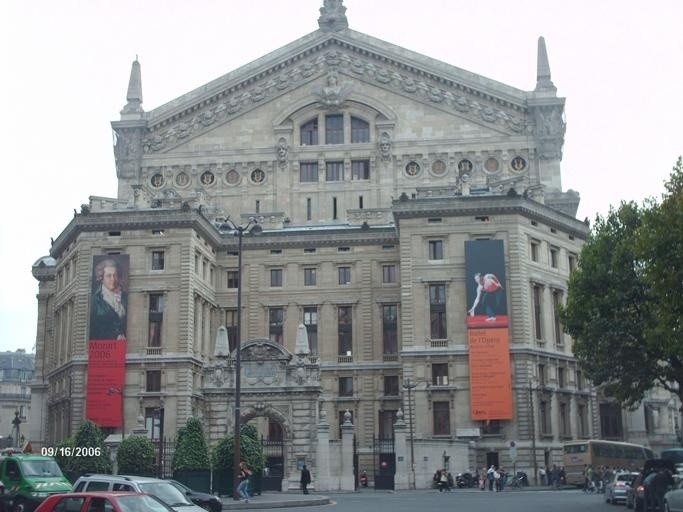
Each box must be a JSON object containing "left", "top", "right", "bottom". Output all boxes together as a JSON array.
[{"left": 1, "top": 450, "right": 73, "bottom": 512}]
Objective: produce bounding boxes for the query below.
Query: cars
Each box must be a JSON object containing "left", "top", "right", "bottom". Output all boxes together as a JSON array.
[
  {"left": 34, "top": 490, "right": 174, "bottom": 511},
  {"left": 627, "top": 448, "right": 683, "bottom": 511},
  {"left": 163, "top": 478, "right": 222, "bottom": 512},
  {"left": 604, "top": 472, "right": 639, "bottom": 505}
]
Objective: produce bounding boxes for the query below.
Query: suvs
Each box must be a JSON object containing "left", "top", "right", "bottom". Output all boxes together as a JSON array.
[{"left": 72, "top": 473, "right": 209, "bottom": 512}]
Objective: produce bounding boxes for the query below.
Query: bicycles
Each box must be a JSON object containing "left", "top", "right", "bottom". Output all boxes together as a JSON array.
[{"left": 509, "top": 477, "right": 524, "bottom": 489}]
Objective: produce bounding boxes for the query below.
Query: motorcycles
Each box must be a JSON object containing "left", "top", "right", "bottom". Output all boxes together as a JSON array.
[{"left": 360, "top": 471, "right": 368, "bottom": 487}]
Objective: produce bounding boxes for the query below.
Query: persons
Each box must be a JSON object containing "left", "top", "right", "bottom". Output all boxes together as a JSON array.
[
  {"left": 380, "top": 136, "right": 392, "bottom": 152},
  {"left": 440, "top": 468, "right": 451, "bottom": 492},
  {"left": 300, "top": 465, "right": 311, "bottom": 494},
  {"left": 487, "top": 464, "right": 496, "bottom": 491},
  {"left": 468, "top": 271, "right": 505, "bottom": 322},
  {"left": 278, "top": 142, "right": 288, "bottom": 157},
  {"left": 323, "top": 71, "right": 342, "bottom": 95},
  {"left": 496, "top": 465, "right": 506, "bottom": 490},
  {"left": 642, "top": 472, "right": 657, "bottom": 512},
  {"left": 480, "top": 467, "right": 487, "bottom": 490},
  {"left": 90, "top": 258, "right": 127, "bottom": 341},
  {"left": 496, "top": 471, "right": 502, "bottom": 492},
  {"left": 236, "top": 461, "right": 253, "bottom": 502},
  {"left": 656, "top": 467, "right": 675, "bottom": 512},
  {"left": 581, "top": 463, "right": 644, "bottom": 495},
  {"left": 539, "top": 464, "right": 566, "bottom": 486}
]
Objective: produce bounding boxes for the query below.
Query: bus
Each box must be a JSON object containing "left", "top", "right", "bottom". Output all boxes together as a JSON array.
[{"left": 561, "top": 440, "right": 658, "bottom": 486}]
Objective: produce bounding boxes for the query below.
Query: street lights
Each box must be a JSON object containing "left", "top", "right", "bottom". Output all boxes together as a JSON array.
[
  {"left": 528, "top": 376, "right": 542, "bottom": 484},
  {"left": 402, "top": 378, "right": 418, "bottom": 489},
  {"left": 219, "top": 217, "right": 263, "bottom": 500}
]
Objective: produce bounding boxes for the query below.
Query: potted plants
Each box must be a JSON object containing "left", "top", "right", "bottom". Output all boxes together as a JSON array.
[{"left": 113, "top": 415, "right": 263, "bottom": 498}]
